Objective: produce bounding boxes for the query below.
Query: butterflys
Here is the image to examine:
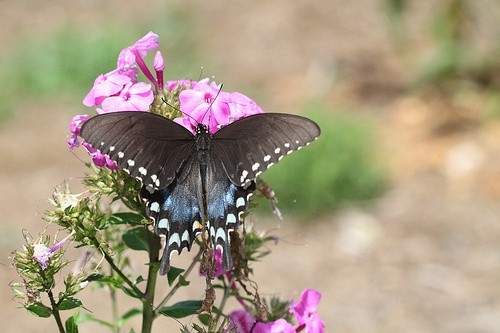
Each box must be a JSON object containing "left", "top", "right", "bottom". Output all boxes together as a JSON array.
[{"left": 80, "top": 83, "right": 321, "bottom": 276}]
